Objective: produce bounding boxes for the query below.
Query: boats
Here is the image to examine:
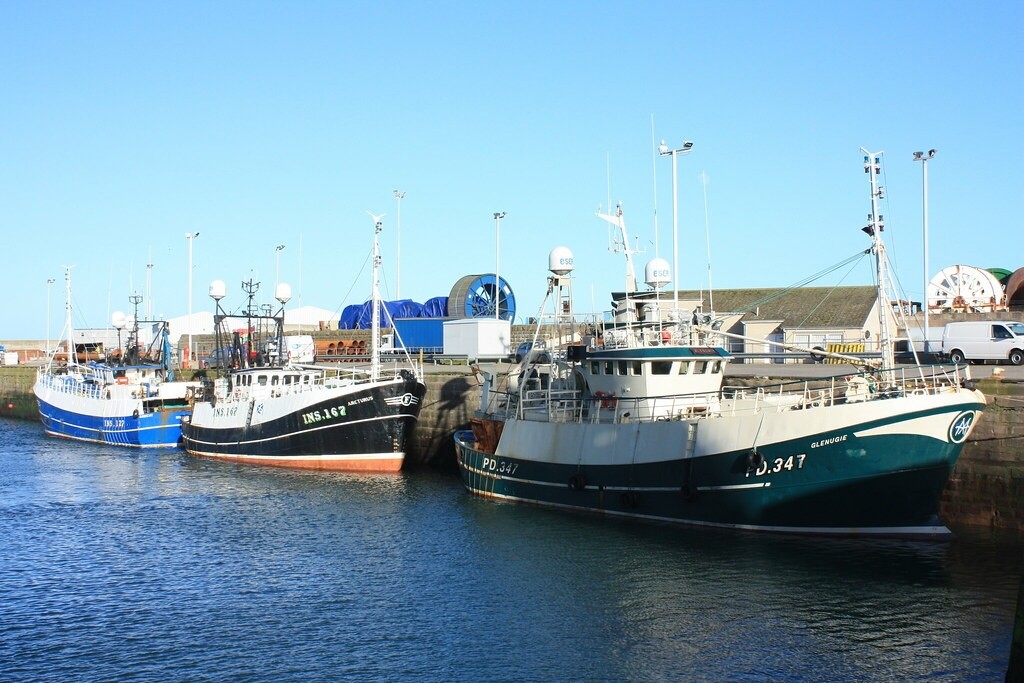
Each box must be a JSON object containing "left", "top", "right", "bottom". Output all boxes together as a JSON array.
[
  {"left": 34, "top": 260, "right": 211, "bottom": 448},
  {"left": 449, "top": 116, "right": 990, "bottom": 542},
  {"left": 176, "top": 211, "right": 427, "bottom": 473}
]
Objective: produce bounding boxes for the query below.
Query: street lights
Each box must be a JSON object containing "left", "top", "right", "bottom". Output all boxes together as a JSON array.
[
  {"left": 185, "top": 231, "right": 199, "bottom": 362},
  {"left": 659, "top": 139, "right": 694, "bottom": 317},
  {"left": 45, "top": 278, "right": 56, "bottom": 362},
  {"left": 144, "top": 262, "right": 154, "bottom": 352},
  {"left": 491, "top": 211, "right": 507, "bottom": 320},
  {"left": 275, "top": 244, "right": 286, "bottom": 317},
  {"left": 912, "top": 149, "right": 938, "bottom": 365}
]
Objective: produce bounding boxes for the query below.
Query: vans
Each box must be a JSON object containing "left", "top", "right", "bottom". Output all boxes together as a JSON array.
[{"left": 942, "top": 321, "right": 1024, "bottom": 367}]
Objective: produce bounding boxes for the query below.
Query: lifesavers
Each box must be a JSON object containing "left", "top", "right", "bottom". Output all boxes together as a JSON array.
[{"left": 592, "top": 390, "right": 608, "bottom": 408}]
